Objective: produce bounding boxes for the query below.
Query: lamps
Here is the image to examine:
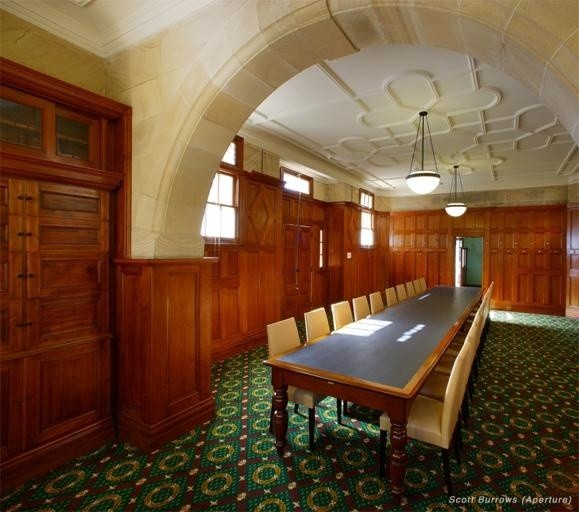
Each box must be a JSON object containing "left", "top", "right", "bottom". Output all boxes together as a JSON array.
[
  {"left": 405, "top": 111, "right": 440, "bottom": 194},
  {"left": 445, "top": 165, "right": 468, "bottom": 218}
]
